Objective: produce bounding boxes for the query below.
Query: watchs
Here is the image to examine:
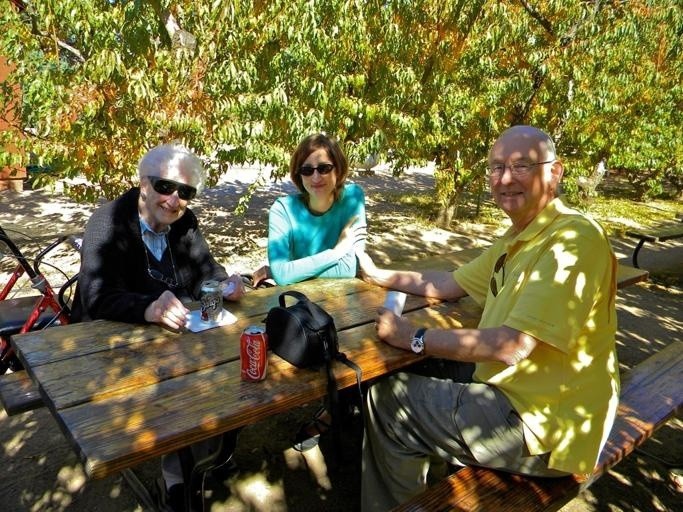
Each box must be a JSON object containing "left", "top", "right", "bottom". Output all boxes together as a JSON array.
[{"left": 410, "top": 327, "right": 428, "bottom": 356}]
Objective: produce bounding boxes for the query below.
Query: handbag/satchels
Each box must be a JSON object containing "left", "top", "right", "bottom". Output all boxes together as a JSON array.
[{"left": 260, "top": 291, "right": 337, "bottom": 373}]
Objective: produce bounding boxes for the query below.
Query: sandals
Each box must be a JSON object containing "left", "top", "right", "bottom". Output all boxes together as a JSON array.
[{"left": 289, "top": 406, "right": 332, "bottom": 452}]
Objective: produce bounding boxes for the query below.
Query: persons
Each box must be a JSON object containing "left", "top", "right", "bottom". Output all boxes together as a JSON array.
[
  {"left": 70, "top": 143, "right": 245, "bottom": 512},
  {"left": 356, "top": 125, "right": 622, "bottom": 512},
  {"left": 251, "top": 132, "right": 368, "bottom": 452}
]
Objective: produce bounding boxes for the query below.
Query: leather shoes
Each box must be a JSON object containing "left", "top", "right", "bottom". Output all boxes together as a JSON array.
[{"left": 164, "top": 455, "right": 189, "bottom": 512}]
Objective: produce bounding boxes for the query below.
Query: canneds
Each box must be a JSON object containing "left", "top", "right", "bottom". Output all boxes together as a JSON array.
[
  {"left": 200, "top": 279, "right": 223, "bottom": 324},
  {"left": 240, "top": 326, "right": 268, "bottom": 383}
]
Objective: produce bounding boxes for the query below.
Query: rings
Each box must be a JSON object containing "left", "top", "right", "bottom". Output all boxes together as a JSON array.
[
  {"left": 374, "top": 322, "right": 377, "bottom": 329},
  {"left": 162, "top": 309, "right": 167, "bottom": 317}
]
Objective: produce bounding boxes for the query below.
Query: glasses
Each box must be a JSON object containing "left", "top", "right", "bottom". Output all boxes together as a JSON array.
[
  {"left": 147, "top": 176, "right": 196, "bottom": 200},
  {"left": 485, "top": 159, "right": 556, "bottom": 176},
  {"left": 145, "top": 248, "right": 179, "bottom": 289},
  {"left": 299, "top": 164, "right": 336, "bottom": 176}
]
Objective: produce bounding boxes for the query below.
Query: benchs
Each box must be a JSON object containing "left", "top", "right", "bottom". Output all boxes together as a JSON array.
[
  {"left": 625, "top": 221, "right": 683, "bottom": 268},
  {"left": 388, "top": 341, "right": 682, "bottom": 512},
  {"left": 0, "top": 369, "right": 44, "bottom": 418}
]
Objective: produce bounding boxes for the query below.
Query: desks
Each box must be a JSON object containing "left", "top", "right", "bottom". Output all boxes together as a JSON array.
[{"left": 11, "top": 243, "right": 650, "bottom": 512}]
